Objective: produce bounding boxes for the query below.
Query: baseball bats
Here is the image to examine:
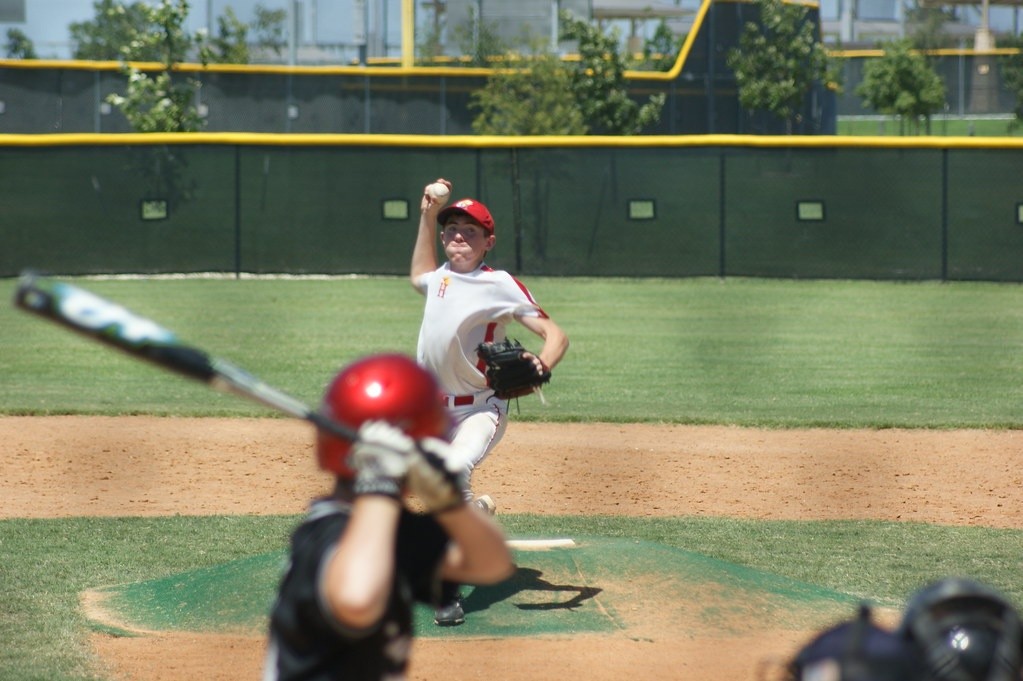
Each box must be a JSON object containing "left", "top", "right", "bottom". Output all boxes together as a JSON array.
[{"left": 9, "top": 265, "right": 446, "bottom": 472}]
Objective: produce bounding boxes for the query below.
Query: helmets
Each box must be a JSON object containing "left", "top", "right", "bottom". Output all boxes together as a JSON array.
[
  {"left": 317, "top": 354, "right": 453, "bottom": 475},
  {"left": 787, "top": 573, "right": 1023, "bottom": 680}
]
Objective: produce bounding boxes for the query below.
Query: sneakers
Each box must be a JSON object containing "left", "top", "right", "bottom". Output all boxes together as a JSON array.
[
  {"left": 434, "top": 602, "right": 465, "bottom": 625},
  {"left": 473, "top": 495, "right": 496, "bottom": 517}
]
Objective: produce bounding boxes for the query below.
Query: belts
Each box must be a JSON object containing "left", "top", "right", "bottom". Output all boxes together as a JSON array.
[{"left": 442, "top": 395, "right": 475, "bottom": 407}]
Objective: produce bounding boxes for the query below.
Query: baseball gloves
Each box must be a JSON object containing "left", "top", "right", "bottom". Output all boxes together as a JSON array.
[
  {"left": 473, "top": 336, "right": 552, "bottom": 416},
  {"left": 757, "top": 600, "right": 903, "bottom": 680}
]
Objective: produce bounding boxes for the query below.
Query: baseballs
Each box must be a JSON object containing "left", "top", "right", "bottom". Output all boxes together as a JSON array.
[{"left": 429, "top": 182, "right": 450, "bottom": 204}]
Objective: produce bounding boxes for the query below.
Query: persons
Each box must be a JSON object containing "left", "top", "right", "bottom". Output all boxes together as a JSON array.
[
  {"left": 409, "top": 178, "right": 570, "bottom": 627},
  {"left": 264, "top": 352, "right": 516, "bottom": 681},
  {"left": 782, "top": 578, "right": 1023, "bottom": 681}
]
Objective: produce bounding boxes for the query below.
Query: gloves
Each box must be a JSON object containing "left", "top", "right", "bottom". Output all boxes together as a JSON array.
[
  {"left": 410, "top": 437, "right": 467, "bottom": 514},
  {"left": 343, "top": 420, "right": 414, "bottom": 500}
]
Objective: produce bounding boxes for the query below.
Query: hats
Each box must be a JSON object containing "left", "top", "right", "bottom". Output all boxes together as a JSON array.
[{"left": 437, "top": 200, "right": 495, "bottom": 234}]
